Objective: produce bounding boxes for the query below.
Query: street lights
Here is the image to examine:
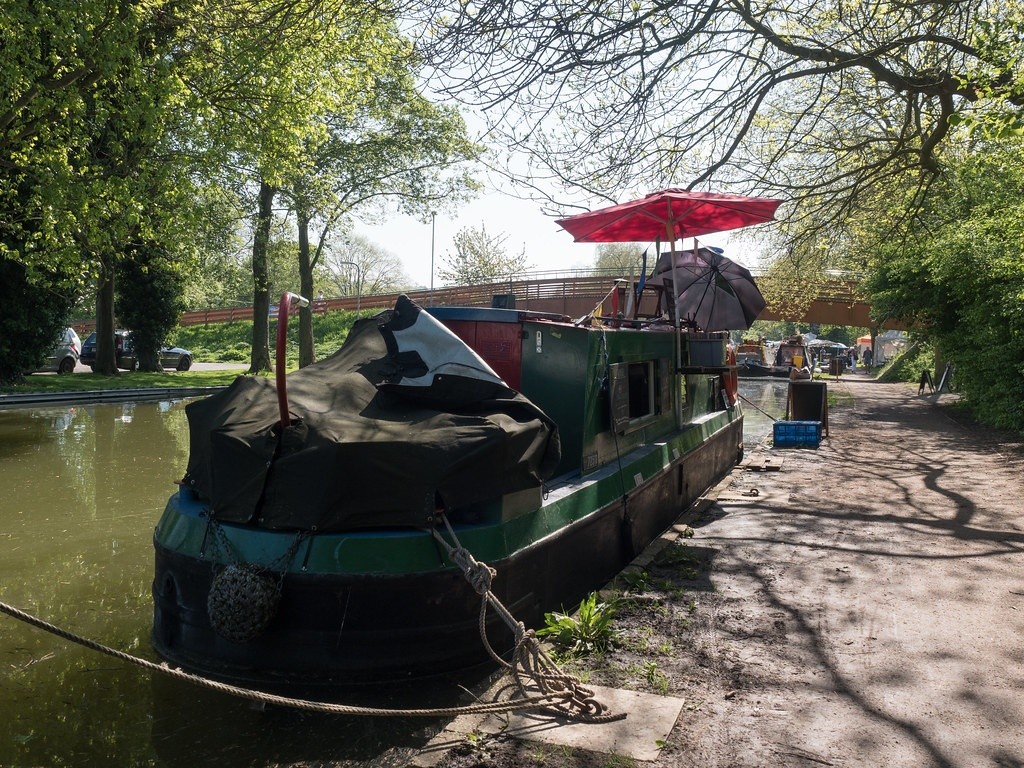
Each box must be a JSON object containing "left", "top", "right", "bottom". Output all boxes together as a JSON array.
[
  {"left": 340, "top": 262, "right": 360, "bottom": 320},
  {"left": 431, "top": 211, "right": 438, "bottom": 309}
]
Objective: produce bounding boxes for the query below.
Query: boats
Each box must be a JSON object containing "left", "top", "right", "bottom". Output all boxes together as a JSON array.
[
  {"left": 731, "top": 333, "right": 850, "bottom": 377},
  {"left": 151, "top": 294, "right": 747, "bottom": 705}
]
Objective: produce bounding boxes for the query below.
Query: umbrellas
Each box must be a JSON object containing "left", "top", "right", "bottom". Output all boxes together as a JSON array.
[
  {"left": 553, "top": 188, "right": 788, "bottom": 432},
  {"left": 645, "top": 248, "right": 768, "bottom": 332}
]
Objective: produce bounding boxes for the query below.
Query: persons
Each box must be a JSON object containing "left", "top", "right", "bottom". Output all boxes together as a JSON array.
[
  {"left": 820, "top": 346, "right": 826, "bottom": 362},
  {"left": 850, "top": 345, "right": 872, "bottom": 374}
]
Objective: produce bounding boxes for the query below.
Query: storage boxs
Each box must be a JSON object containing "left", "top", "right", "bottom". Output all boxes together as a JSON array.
[{"left": 773, "top": 421, "right": 823, "bottom": 447}]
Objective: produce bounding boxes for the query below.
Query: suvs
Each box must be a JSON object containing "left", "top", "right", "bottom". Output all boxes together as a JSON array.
[{"left": 79, "top": 329, "right": 194, "bottom": 372}]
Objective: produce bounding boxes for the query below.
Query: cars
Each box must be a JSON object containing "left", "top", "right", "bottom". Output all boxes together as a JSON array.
[{"left": 23, "top": 326, "right": 81, "bottom": 376}]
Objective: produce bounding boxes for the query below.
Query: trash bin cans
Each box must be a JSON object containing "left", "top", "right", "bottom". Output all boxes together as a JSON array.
[{"left": 829, "top": 358, "right": 843, "bottom": 375}]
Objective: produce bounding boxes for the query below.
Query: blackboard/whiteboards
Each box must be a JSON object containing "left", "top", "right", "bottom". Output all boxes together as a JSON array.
[
  {"left": 919, "top": 369, "right": 934, "bottom": 390},
  {"left": 787, "top": 382, "right": 829, "bottom": 430}
]
